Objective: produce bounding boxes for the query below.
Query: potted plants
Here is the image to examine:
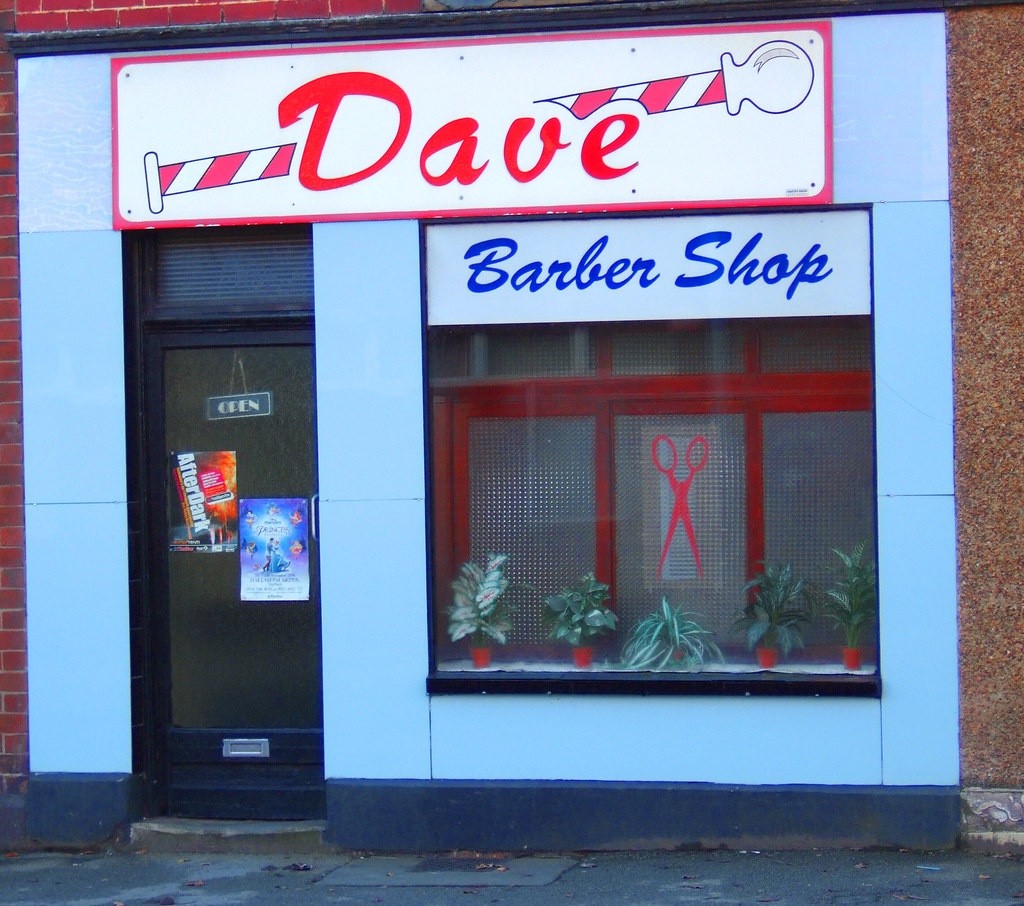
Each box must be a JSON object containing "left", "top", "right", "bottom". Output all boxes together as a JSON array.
[
  {"left": 727, "top": 561, "right": 818, "bottom": 670},
  {"left": 620, "top": 596, "right": 725, "bottom": 672},
  {"left": 541, "top": 572, "right": 618, "bottom": 667},
  {"left": 818, "top": 539, "right": 877, "bottom": 670},
  {"left": 440, "top": 552, "right": 535, "bottom": 667}
]
